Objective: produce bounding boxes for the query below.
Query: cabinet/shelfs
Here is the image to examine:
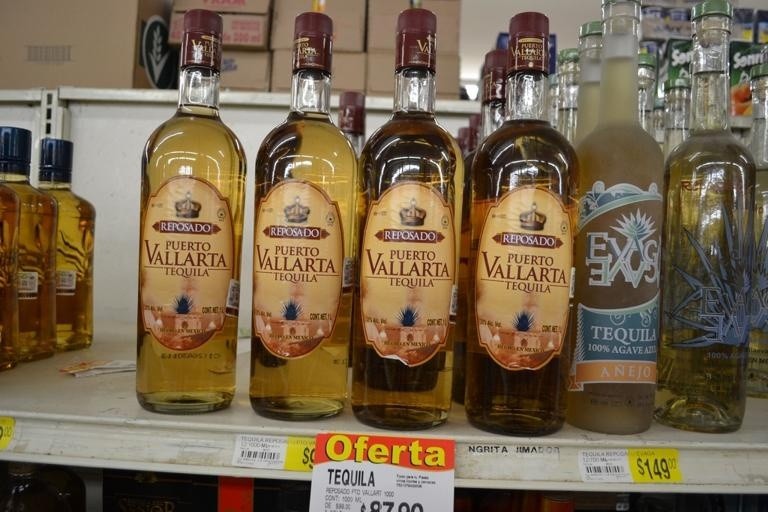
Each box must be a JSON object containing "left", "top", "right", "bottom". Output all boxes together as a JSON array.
[{"left": 0, "top": 86, "right": 768, "bottom": 496}]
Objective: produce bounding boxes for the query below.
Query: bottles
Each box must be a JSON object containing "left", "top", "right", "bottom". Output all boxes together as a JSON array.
[
  {"left": 0, "top": 457, "right": 87, "bottom": 511},
  {"left": 0, "top": 124, "right": 95, "bottom": 369},
  {"left": 134, "top": 8, "right": 249, "bottom": 416},
  {"left": 248, "top": 1, "right": 767, "bottom": 438}
]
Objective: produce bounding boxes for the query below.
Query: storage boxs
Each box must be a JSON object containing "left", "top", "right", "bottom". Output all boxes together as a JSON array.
[
  {"left": 270, "top": 53, "right": 366, "bottom": 90},
  {"left": 1, "top": 1, "right": 177, "bottom": 89},
  {"left": 222, "top": 50, "right": 270, "bottom": 89},
  {"left": 268, "top": 1, "right": 367, "bottom": 49},
  {"left": 170, "top": 0, "right": 274, "bottom": 49},
  {"left": 367, "top": 1, "right": 463, "bottom": 52},
  {"left": 365, "top": 54, "right": 461, "bottom": 93}
]
